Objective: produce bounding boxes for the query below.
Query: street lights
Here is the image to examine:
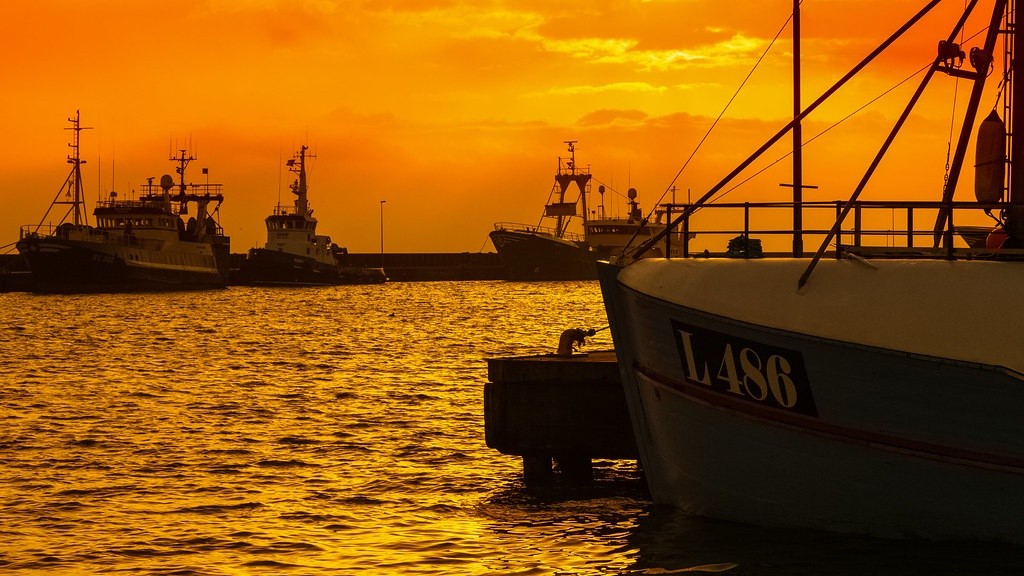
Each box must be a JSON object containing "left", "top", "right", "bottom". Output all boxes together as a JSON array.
[{"left": 380, "top": 200, "right": 386, "bottom": 253}]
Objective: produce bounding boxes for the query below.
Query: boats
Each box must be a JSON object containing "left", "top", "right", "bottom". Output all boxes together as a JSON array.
[
  {"left": 229, "top": 145, "right": 346, "bottom": 285},
  {"left": 597, "top": 2, "right": 1024, "bottom": 576},
  {"left": 344, "top": 266, "right": 386, "bottom": 284},
  {"left": 487, "top": 141, "right": 681, "bottom": 278},
  {"left": 18, "top": 137, "right": 232, "bottom": 287}
]
